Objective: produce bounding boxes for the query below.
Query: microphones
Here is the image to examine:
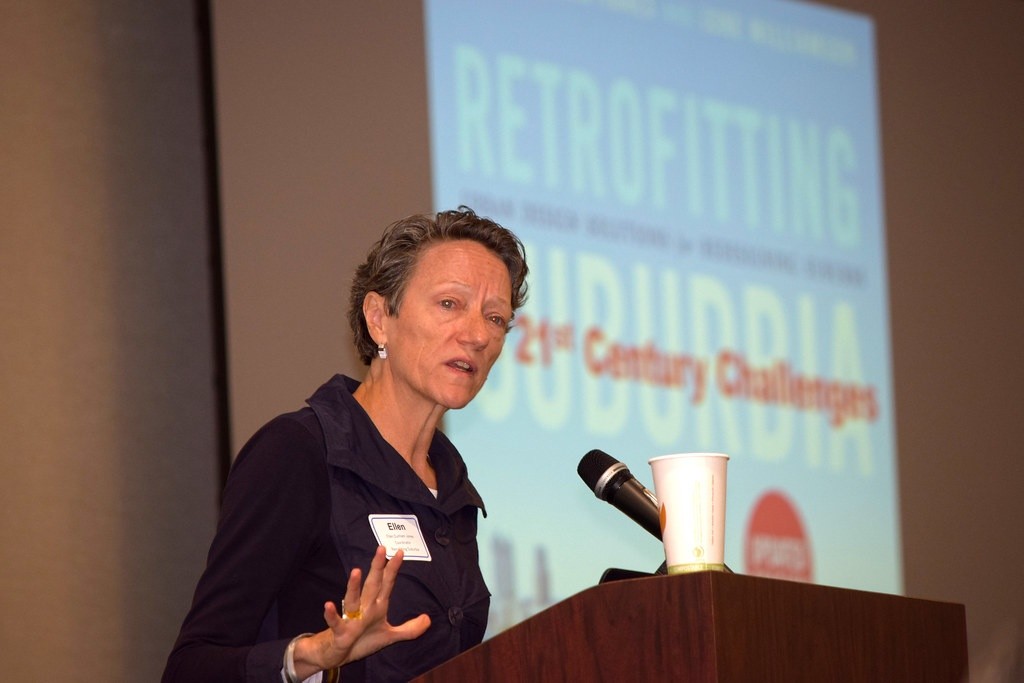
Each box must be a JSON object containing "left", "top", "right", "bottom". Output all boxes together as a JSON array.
[{"left": 575, "top": 449, "right": 662, "bottom": 544}]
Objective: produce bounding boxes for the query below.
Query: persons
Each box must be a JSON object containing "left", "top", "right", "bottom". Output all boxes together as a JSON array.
[{"left": 161, "top": 206, "right": 530, "bottom": 683}]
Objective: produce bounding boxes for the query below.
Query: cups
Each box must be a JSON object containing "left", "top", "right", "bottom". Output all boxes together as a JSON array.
[{"left": 648, "top": 453, "right": 728, "bottom": 575}]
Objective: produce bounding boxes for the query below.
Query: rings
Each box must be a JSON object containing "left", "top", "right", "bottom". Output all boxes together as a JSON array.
[{"left": 341, "top": 600, "right": 360, "bottom": 619}]
